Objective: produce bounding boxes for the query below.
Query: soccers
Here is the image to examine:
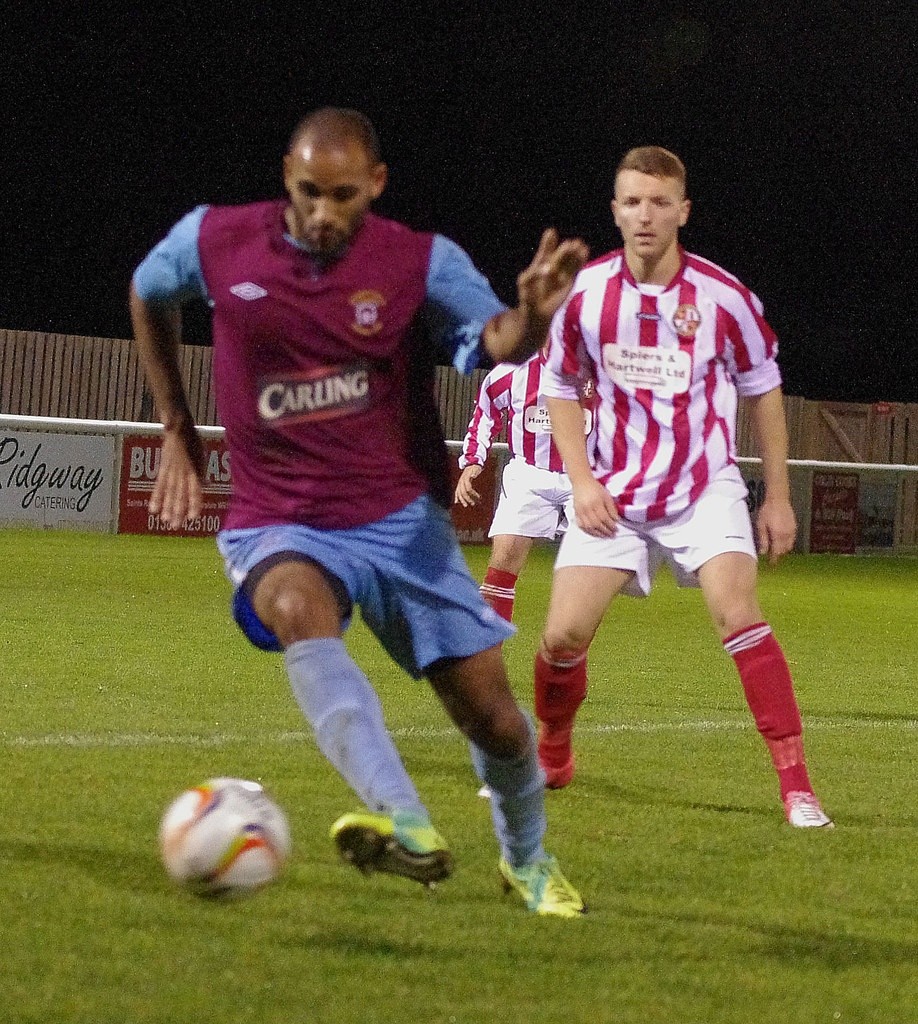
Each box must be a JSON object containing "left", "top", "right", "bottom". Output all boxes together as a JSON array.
[{"left": 161, "top": 777, "right": 293, "bottom": 902}]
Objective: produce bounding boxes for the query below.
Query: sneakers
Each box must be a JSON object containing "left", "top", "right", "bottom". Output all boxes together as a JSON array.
[
  {"left": 497, "top": 846, "right": 584, "bottom": 918},
  {"left": 330, "top": 813, "right": 455, "bottom": 884},
  {"left": 784, "top": 793, "right": 836, "bottom": 829},
  {"left": 538, "top": 757, "right": 573, "bottom": 790}
]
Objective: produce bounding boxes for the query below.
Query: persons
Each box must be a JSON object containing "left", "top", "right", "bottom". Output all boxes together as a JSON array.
[
  {"left": 453, "top": 326, "right": 600, "bottom": 622},
  {"left": 477, "top": 145, "right": 839, "bottom": 829},
  {"left": 129, "top": 103, "right": 593, "bottom": 917}
]
